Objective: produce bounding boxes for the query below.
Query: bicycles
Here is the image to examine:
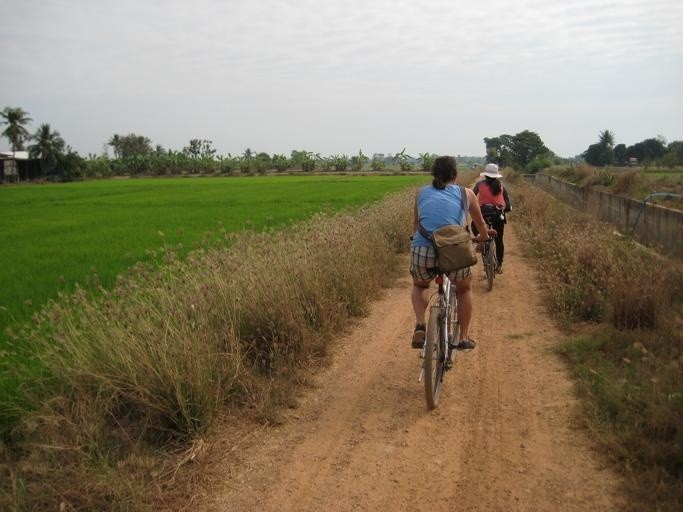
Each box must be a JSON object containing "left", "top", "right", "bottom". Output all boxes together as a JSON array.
[
  {"left": 466, "top": 204, "right": 510, "bottom": 292},
  {"left": 403, "top": 231, "right": 492, "bottom": 411}
]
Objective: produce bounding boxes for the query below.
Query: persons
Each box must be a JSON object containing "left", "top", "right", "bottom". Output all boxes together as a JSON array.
[
  {"left": 465, "top": 163, "right": 511, "bottom": 274},
  {"left": 408, "top": 156, "right": 477, "bottom": 350}
]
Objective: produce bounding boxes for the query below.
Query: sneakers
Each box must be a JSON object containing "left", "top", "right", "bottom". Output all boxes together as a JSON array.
[
  {"left": 458, "top": 339, "right": 475, "bottom": 353},
  {"left": 412, "top": 325, "right": 426, "bottom": 348}
]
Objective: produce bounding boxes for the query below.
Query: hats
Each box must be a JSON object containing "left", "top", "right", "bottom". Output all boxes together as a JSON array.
[{"left": 478, "top": 164, "right": 503, "bottom": 179}]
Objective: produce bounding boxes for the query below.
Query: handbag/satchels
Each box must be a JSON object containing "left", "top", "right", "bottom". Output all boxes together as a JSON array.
[
  {"left": 432, "top": 224, "right": 476, "bottom": 271},
  {"left": 482, "top": 203, "right": 503, "bottom": 216}
]
